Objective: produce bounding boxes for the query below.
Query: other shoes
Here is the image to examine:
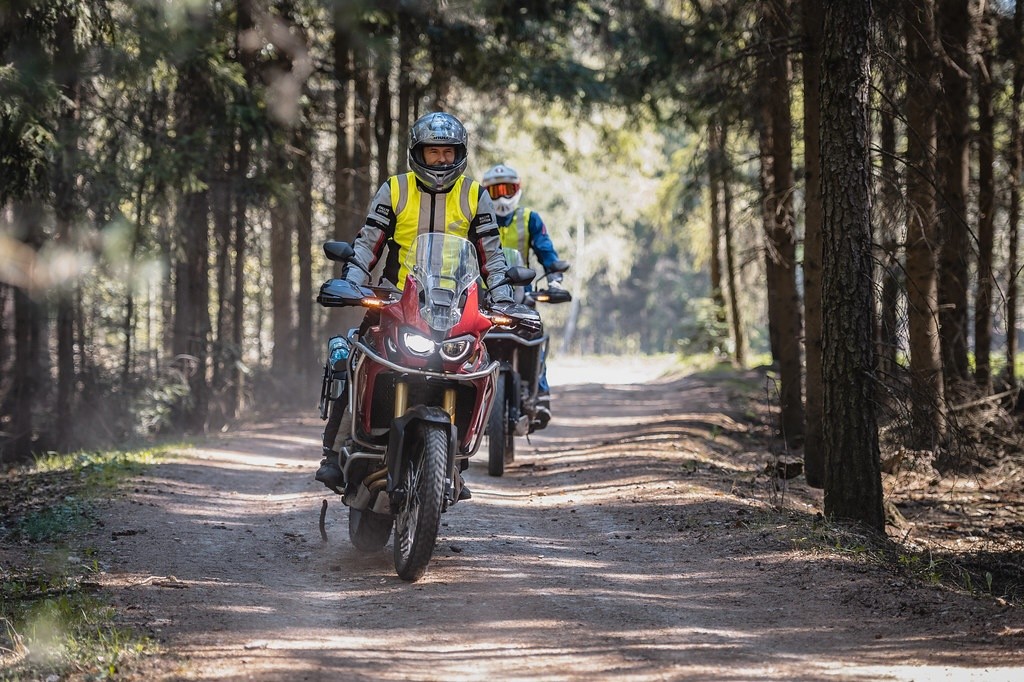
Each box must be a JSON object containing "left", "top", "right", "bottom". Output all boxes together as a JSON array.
[
  {"left": 454, "top": 483, "right": 471, "bottom": 500},
  {"left": 315, "top": 451, "right": 344, "bottom": 485},
  {"left": 531, "top": 392, "right": 550, "bottom": 426}
]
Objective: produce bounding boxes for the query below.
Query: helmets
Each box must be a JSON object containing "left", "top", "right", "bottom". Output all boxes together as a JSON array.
[
  {"left": 482, "top": 165, "right": 522, "bottom": 217},
  {"left": 406, "top": 112, "right": 468, "bottom": 192}
]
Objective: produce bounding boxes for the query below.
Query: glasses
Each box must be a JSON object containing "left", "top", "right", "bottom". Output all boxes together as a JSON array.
[{"left": 486, "top": 183, "right": 519, "bottom": 200}]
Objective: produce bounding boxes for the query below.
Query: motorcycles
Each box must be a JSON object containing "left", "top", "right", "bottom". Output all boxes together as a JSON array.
[
  {"left": 315, "top": 230, "right": 543, "bottom": 583},
  {"left": 478, "top": 259, "right": 571, "bottom": 477}
]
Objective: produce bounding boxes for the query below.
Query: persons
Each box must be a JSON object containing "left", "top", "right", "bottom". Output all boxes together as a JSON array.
[
  {"left": 482, "top": 165, "right": 563, "bottom": 429},
  {"left": 315, "top": 112, "right": 514, "bottom": 500}
]
